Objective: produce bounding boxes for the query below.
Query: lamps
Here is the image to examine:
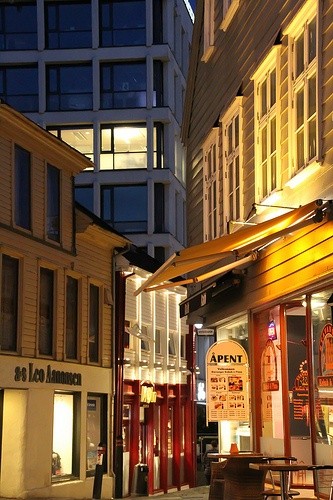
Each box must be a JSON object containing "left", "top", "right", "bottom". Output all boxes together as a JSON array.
[
  {"left": 139, "top": 378, "right": 156, "bottom": 410},
  {"left": 225, "top": 219, "right": 261, "bottom": 235},
  {"left": 243, "top": 202, "right": 299, "bottom": 222}
]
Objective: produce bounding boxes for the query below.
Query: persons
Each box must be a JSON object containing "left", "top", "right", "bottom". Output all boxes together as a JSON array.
[{"left": 201, "top": 440, "right": 219, "bottom": 486}]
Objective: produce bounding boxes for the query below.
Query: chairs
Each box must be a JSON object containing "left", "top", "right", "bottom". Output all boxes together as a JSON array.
[{"left": 259, "top": 456, "right": 301, "bottom": 500}]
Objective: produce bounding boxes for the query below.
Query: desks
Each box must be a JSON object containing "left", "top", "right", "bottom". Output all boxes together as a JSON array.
[{"left": 247, "top": 463, "right": 333, "bottom": 500}]
